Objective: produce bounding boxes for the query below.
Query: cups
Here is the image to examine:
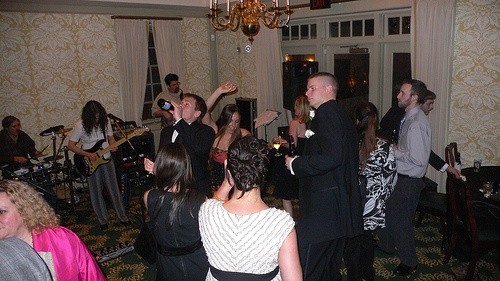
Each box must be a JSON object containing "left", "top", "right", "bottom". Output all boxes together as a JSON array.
[
  {"left": 483, "top": 181, "right": 493, "bottom": 199},
  {"left": 473, "top": 159, "right": 482, "bottom": 172}
]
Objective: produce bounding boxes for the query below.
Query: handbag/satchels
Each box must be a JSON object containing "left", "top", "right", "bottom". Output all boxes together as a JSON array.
[{"left": 133, "top": 219, "right": 159, "bottom": 266}]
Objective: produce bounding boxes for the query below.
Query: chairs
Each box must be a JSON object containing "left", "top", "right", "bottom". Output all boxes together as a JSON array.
[
  {"left": 442, "top": 142, "right": 500, "bottom": 281},
  {"left": 417, "top": 176, "right": 450, "bottom": 250}
]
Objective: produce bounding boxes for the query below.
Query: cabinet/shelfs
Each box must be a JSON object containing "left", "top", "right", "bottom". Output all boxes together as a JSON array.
[{"left": 113, "top": 132, "right": 156, "bottom": 196}]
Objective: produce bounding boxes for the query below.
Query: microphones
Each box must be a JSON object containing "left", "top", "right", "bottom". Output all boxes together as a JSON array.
[{"left": 108, "top": 114, "right": 122, "bottom": 121}]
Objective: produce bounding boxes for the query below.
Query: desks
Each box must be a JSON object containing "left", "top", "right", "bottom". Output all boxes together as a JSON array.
[{"left": 447, "top": 166, "right": 500, "bottom": 259}]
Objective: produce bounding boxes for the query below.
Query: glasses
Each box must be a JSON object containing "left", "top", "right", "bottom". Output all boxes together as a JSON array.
[{"left": 169, "top": 82, "right": 181, "bottom": 86}]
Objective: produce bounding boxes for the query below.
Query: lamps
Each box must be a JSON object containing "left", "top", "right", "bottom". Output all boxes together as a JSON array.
[{"left": 208, "top": 0, "right": 295, "bottom": 45}]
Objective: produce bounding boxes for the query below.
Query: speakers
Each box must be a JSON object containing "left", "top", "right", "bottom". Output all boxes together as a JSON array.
[{"left": 282, "top": 61, "right": 318, "bottom": 111}]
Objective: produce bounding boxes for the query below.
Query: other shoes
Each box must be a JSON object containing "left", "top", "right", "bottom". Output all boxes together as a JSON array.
[
  {"left": 394, "top": 262, "right": 419, "bottom": 276},
  {"left": 121, "top": 216, "right": 132, "bottom": 227},
  {"left": 372, "top": 238, "right": 391, "bottom": 254},
  {"left": 99, "top": 220, "right": 109, "bottom": 230}
]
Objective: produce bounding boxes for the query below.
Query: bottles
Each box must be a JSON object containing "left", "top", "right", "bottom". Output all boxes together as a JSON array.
[
  {"left": 158, "top": 99, "right": 173, "bottom": 111},
  {"left": 288, "top": 135, "right": 297, "bottom": 158}
]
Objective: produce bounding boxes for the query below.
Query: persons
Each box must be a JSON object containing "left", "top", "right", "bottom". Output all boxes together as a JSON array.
[
  {"left": 197, "top": 133, "right": 304, "bottom": 281},
  {"left": 0, "top": 115, "right": 42, "bottom": 180},
  {"left": 371, "top": 79, "right": 432, "bottom": 276},
  {"left": 0, "top": 177, "right": 107, "bottom": 281},
  {"left": 345, "top": 100, "right": 398, "bottom": 281},
  {"left": 378, "top": 89, "right": 462, "bottom": 180},
  {"left": 143, "top": 143, "right": 209, "bottom": 281},
  {"left": 0, "top": 237, "right": 53, "bottom": 281},
  {"left": 284, "top": 72, "right": 365, "bottom": 281},
  {"left": 152, "top": 74, "right": 254, "bottom": 198},
  {"left": 67, "top": 100, "right": 132, "bottom": 230},
  {"left": 275, "top": 96, "right": 318, "bottom": 216}
]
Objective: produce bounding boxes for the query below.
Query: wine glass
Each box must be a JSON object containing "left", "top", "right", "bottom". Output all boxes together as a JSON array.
[{"left": 273, "top": 136, "right": 282, "bottom": 157}]
